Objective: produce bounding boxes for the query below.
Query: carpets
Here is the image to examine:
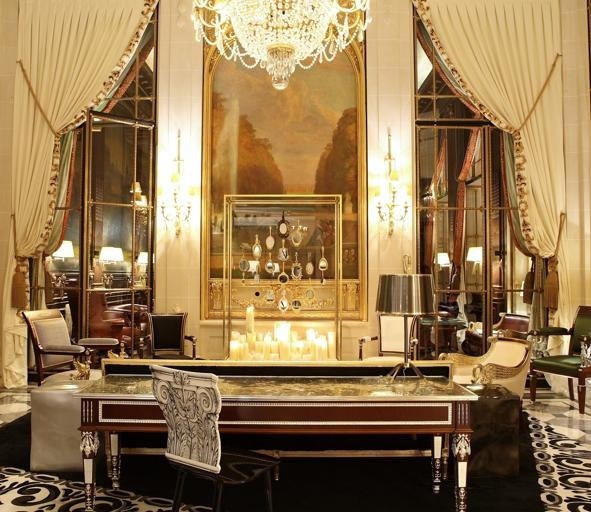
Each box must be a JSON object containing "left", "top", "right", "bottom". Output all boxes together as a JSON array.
[
  {"left": 527, "top": 413, "right": 590, "bottom": 512},
  {"left": 0, "top": 466, "right": 216, "bottom": 512}
]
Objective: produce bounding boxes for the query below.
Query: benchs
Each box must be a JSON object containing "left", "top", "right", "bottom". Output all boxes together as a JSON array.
[{"left": 99, "top": 359, "right": 454, "bottom": 478}]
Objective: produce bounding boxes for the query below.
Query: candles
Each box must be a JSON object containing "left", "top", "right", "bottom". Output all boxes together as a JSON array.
[{"left": 229, "top": 305, "right": 337, "bottom": 364}]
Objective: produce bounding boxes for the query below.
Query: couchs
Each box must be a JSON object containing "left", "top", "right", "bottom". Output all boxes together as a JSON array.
[
  {"left": 462, "top": 314, "right": 531, "bottom": 357},
  {"left": 437, "top": 336, "right": 532, "bottom": 403}
]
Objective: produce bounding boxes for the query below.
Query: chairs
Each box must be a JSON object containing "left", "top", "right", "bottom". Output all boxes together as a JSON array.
[
  {"left": 527, "top": 306, "right": 591, "bottom": 413},
  {"left": 150, "top": 364, "right": 281, "bottom": 512},
  {"left": 20, "top": 308, "right": 119, "bottom": 387},
  {"left": 138, "top": 311, "right": 201, "bottom": 360}
]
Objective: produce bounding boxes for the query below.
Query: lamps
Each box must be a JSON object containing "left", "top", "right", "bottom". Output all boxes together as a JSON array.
[
  {"left": 433, "top": 250, "right": 450, "bottom": 270},
  {"left": 370, "top": 169, "right": 409, "bottom": 237},
  {"left": 375, "top": 273, "right": 435, "bottom": 387},
  {"left": 136, "top": 251, "right": 149, "bottom": 285},
  {"left": 191, "top": 0, "right": 371, "bottom": 90},
  {"left": 130, "top": 181, "right": 142, "bottom": 201},
  {"left": 135, "top": 195, "right": 148, "bottom": 214},
  {"left": 114, "top": 246, "right": 124, "bottom": 264},
  {"left": 467, "top": 247, "right": 483, "bottom": 288},
  {"left": 98, "top": 246, "right": 114, "bottom": 264},
  {"left": 52, "top": 240, "right": 74, "bottom": 265},
  {"left": 158, "top": 174, "right": 197, "bottom": 239}
]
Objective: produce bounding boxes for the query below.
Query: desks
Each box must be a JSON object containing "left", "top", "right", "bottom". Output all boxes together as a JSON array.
[{"left": 73, "top": 374, "right": 479, "bottom": 512}]
